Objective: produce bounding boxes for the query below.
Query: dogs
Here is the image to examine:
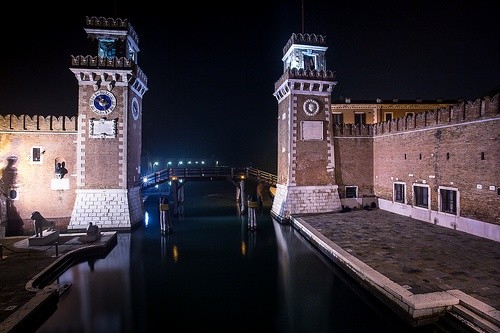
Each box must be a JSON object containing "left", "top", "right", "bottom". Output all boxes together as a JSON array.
[{"left": 31, "top": 212, "right": 56, "bottom": 238}]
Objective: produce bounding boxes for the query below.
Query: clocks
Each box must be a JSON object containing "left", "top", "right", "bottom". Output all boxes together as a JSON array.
[{"left": 89, "top": 89, "right": 116, "bottom": 116}]
